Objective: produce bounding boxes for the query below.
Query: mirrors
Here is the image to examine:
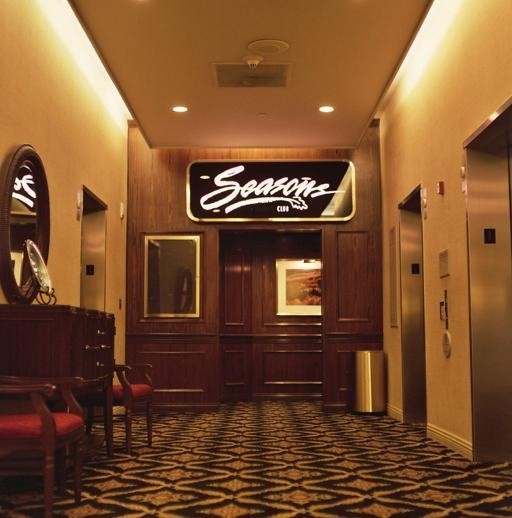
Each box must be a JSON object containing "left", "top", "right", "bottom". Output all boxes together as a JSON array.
[
  {"left": 140, "top": 231, "right": 202, "bottom": 318},
  {"left": 0, "top": 142, "right": 56, "bottom": 305}
]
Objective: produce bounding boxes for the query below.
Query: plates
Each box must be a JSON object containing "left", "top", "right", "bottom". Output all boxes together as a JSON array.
[{"left": 24, "top": 238, "right": 57, "bottom": 296}]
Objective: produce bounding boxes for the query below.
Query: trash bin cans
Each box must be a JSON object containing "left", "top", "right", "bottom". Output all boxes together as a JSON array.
[{"left": 354, "top": 350, "right": 387, "bottom": 413}]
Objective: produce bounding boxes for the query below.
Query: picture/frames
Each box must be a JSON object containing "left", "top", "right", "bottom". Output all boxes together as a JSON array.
[{"left": 275, "top": 257, "right": 322, "bottom": 318}]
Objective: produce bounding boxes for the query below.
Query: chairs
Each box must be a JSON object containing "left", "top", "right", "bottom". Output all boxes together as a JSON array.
[
  {"left": 1, "top": 372, "right": 87, "bottom": 517},
  {"left": 113, "top": 363, "right": 157, "bottom": 453}
]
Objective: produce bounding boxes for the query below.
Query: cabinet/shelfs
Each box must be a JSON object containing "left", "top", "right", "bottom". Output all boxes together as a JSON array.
[{"left": 1, "top": 302, "right": 117, "bottom": 465}]
